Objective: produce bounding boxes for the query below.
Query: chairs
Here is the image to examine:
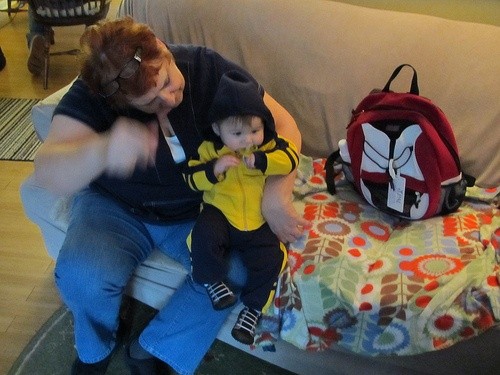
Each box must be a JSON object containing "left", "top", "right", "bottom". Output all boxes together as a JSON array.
[{"left": 26, "top": 0, "right": 106, "bottom": 89}]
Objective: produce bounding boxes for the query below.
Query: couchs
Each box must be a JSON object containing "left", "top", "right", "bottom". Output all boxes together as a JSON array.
[{"left": 19, "top": 0, "right": 500, "bottom": 375}]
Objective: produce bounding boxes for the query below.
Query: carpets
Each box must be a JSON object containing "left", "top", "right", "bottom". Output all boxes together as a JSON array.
[{"left": 0, "top": 96, "right": 45, "bottom": 161}]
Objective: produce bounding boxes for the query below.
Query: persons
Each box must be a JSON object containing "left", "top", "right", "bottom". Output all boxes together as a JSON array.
[
  {"left": 25, "top": 0, "right": 109, "bottom": 77},
  {"left": 33, "top": 14, "right": 314, "bottom": 375},
  {"left": 180, "top": 68, "right": 300, "bottom": 345}
]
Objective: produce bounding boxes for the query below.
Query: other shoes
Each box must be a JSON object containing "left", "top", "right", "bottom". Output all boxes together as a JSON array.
[
  {"left": 124, "top": 342, "right": 159, "bottom": 375},
  {"left": 232, "top": 307, "right": 262, "bottom": 344},
  {"left": 27, "top": 34, "right": 50, "bottom": 74},
  {"left": 69, "top": 346, "right": 115, "bottom": 375},
  {"left": 206, "top": 281, "right": 237, "bottom": 311}
]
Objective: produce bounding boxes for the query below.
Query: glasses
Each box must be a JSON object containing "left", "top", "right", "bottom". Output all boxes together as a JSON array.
[{"left": 97, "top": 45, "right": 143, "bottom": 99}]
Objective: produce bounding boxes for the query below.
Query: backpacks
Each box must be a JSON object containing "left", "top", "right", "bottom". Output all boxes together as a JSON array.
[{"left": 341, "top": 63, "right": 468, "bottom": 219}]
